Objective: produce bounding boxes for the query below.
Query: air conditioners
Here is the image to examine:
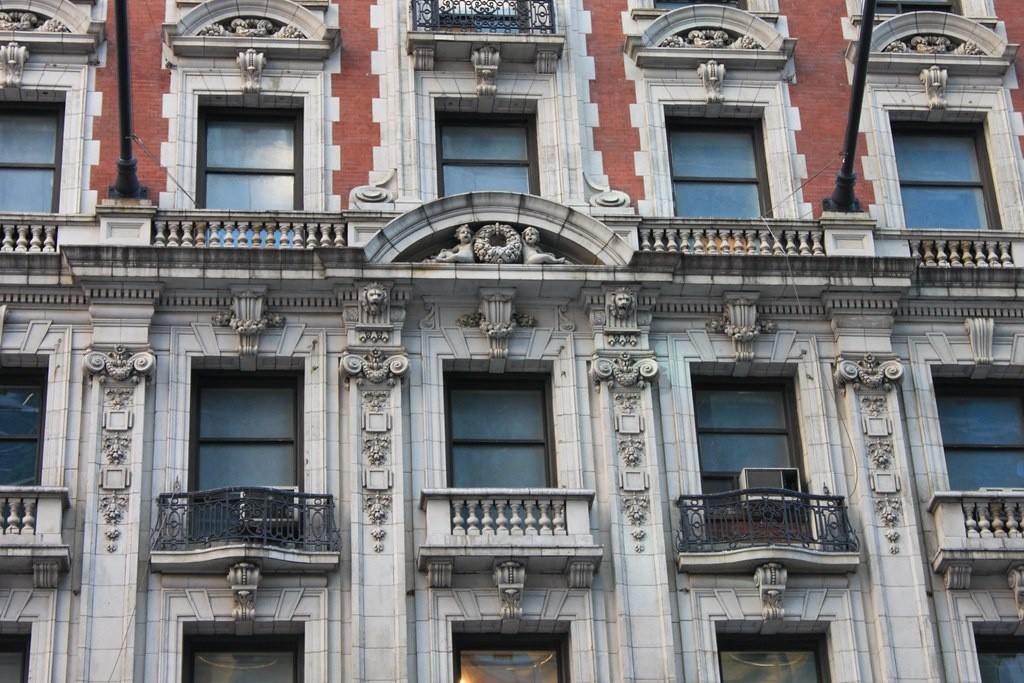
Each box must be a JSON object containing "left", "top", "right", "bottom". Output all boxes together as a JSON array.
[
  {"left": 237, "top": 485, "right": 302, "bottom": 528},
  {"left": 738, "top": 466, "right": 804, "bottom": 509}
]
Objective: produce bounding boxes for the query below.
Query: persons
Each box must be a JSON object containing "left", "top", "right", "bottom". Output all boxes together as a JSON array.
[
  {"left": 520, "top": 225, "right": 571, "bottom": 265},
  {"left": 430, "top": 224, "right": 476, "bottom": 265}
]
